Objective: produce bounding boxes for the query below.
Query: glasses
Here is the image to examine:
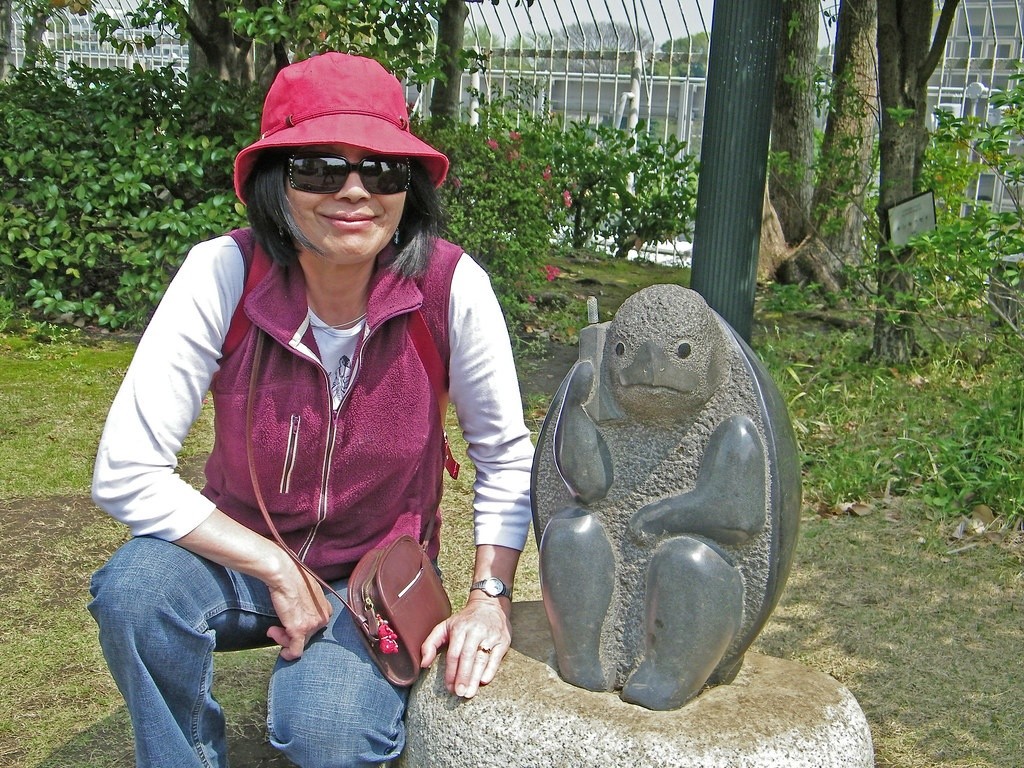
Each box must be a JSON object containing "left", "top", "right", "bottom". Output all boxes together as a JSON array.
[{"left": 284, "top": 152, "right": 413, "bottom": 195}]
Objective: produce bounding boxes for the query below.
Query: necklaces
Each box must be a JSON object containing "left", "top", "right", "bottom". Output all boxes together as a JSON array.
[{"left": 309, "top": 312, "right": 367, "bottom": 328}]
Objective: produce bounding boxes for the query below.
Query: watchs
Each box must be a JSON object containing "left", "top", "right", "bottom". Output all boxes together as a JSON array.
[{"left": 470, "top": 577, "right": 512, "bottom": 601}]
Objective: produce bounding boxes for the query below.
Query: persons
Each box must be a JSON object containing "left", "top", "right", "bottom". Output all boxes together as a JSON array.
[
  {"left": 377, "top": 161, "right": 402, "bottom": 194},
  {"left": 88, "top": 52, "right": 535, "bottom": 768},
  {"left": 322, "top": 162, "right": 335, "bottom": 183}
]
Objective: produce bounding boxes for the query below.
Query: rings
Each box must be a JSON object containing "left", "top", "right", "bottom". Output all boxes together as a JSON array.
[{"left": 478, "top": 645, "right": 490, "bottom": 652}]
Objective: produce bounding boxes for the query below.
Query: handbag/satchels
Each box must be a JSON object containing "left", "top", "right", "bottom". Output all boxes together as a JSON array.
[{"left": 346, "top": 534, "right": 455, "bottom": 688}]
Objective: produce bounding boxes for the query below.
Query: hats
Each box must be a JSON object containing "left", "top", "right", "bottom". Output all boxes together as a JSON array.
[{"left": 234, "top": 53, "right": 449, "bottom": 207}]
